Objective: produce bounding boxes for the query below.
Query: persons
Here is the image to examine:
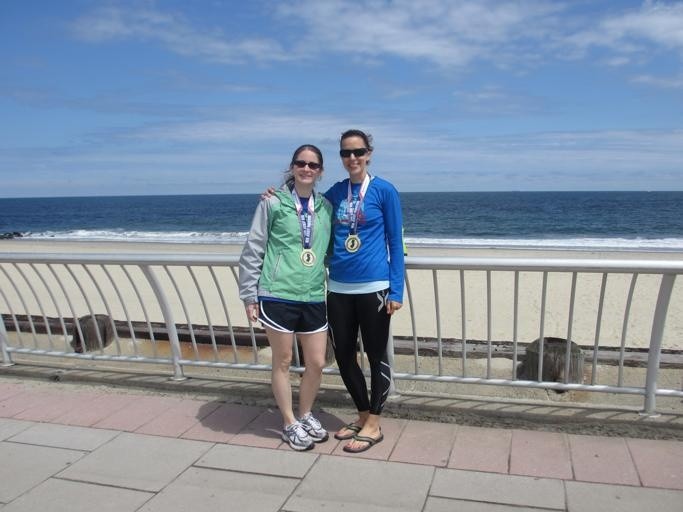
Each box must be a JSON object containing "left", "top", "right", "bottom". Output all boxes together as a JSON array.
[
  {"left": 237, "top": 143, "right": 333, "bottom": 450},
  {"left": 257, "top": 127, "right": 409, "bottom": 454}
]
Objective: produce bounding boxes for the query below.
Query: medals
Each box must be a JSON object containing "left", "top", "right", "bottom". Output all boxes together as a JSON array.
[
  {"left": 343, "top": 234, "right": 362, "bottom": 255},
  {"left": 299, "top": 248, "right": 318, "bottom": 266}
]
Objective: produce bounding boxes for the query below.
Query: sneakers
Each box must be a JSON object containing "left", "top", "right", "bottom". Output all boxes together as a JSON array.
[
  {"left": 295, "top": 412, "right": 327, "bottom": 442},
  {"left": 281, "top": 420, "right": 313, "bottom": 451}
]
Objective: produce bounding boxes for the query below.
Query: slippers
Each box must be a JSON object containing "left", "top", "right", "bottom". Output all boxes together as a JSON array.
[
  {"left": 334, "top": 424, "right": 380, "bottom": 440},
  {"left": 343, "top": 435, "right": 382, "bottom": 453}
]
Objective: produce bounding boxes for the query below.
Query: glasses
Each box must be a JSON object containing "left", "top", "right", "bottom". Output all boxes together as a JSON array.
[
  {"left": 339, "top": 148, "right": 368, "bottom": 157},
  {"left": 293, "top": 160, "right": 320, "bottom": 169}
]
